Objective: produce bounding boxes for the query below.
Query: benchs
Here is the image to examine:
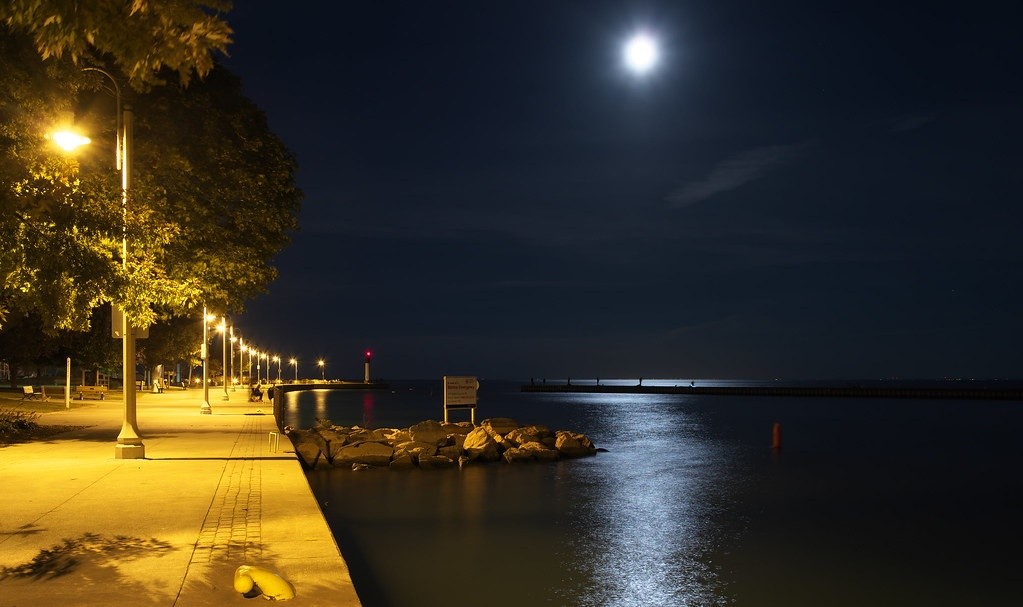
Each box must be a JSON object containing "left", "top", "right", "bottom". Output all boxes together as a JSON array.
[
  {"left": 22, "top": 386, "right": 43, "bottom": 401},
  {"left": 154, "top": 383, "right": 165, "bottom": 394}
]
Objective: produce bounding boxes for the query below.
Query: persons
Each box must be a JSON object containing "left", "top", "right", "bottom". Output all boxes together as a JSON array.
[{"left": 254, "top": 384, "right": 263, "bottom": 400}]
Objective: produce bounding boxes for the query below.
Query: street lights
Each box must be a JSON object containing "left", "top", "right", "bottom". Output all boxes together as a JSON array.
[
  {"left": 227, "top": 325, "right": 235, "bottom": 393},
  {"left": 240, "top": 334, "right": 325, "bottom": 389},
  {"left": 200, "top": 299, "right": 216, "bottom": 415},
  {"left": 218, "top": 311, "right": 231, "bottom": 400},
  {"left": 47, "top": 66, "right": 152, "bottom": 458}
]
